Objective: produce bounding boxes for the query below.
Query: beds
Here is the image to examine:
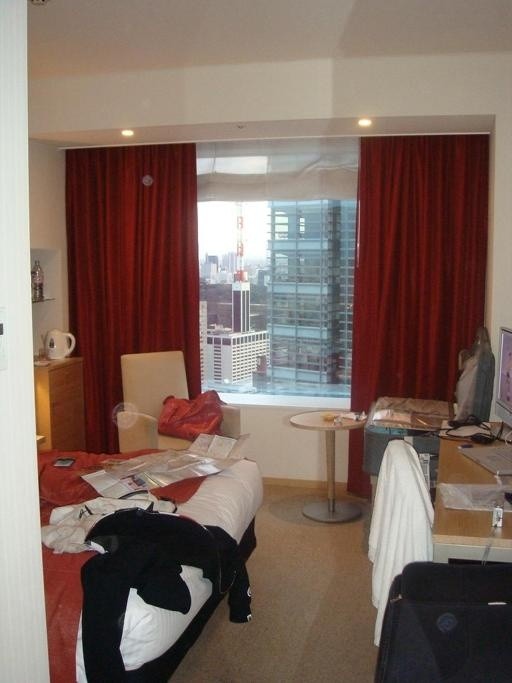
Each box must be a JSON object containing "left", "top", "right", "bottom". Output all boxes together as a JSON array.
[{"left": 37, "top": 452, "right": 264, "bottom": 683}]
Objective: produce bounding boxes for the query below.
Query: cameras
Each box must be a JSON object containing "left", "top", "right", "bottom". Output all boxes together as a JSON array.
[{"left": 491, "top": 499, "right": 504, "bottom": 527}]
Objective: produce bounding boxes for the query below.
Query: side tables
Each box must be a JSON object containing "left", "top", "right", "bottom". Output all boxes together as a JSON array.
[{"left": 290, "top": 411, "right": 367, "bottom": 523}]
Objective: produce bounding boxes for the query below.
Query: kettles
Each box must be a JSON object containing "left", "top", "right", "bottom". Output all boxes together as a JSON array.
[{"left": 41, "top": 330, "right": 75, "bottom": 359}]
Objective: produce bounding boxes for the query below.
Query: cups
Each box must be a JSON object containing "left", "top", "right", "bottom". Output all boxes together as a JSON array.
[{"left": 39, "top": 349, "right": 48, "bottom": 361}]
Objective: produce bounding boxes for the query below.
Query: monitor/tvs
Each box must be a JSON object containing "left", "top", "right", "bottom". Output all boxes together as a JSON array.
[{"left": 495, "top": 326, "right": 512, "bottom": 428}]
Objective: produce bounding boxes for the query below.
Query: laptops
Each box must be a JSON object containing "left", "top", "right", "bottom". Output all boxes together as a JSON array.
[{"left": 461, "top": 446, "right": 512, "bottom": 476}]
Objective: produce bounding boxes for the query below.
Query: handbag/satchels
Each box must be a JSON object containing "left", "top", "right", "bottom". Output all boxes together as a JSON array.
[{"left": 157, "top": 396, "right": 222, "bottom": 440}]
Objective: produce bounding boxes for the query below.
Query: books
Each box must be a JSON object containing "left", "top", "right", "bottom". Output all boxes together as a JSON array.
[{"left": 188, "top": 433, "right": 236, "bottom": 458}]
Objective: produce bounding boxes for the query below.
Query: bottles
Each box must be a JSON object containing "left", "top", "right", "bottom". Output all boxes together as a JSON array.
[{"left": 31, "top": 257, "right": 44, "bottom": 301}]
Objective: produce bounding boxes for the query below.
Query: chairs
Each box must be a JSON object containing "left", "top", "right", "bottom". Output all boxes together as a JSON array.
[
  {"left": 117, "top": 351, "right": 241, "bottom": 453},
  {"left": 366, "top": 439, "right": 435, "bottom": 647}
]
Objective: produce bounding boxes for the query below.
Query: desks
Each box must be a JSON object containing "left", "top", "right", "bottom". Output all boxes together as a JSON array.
[{"left": 431, "top": 438, "right": 512, "bottom": 564}]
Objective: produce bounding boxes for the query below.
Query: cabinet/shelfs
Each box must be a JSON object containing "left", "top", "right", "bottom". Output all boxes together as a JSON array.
[{"left": 36, "top": 357, "right": 86, "bottom": 451}]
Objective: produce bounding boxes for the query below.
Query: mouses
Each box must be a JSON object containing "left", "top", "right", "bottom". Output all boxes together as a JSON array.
[{"left": 471, "top": 433, "right": 494, "bottom": 443}]
